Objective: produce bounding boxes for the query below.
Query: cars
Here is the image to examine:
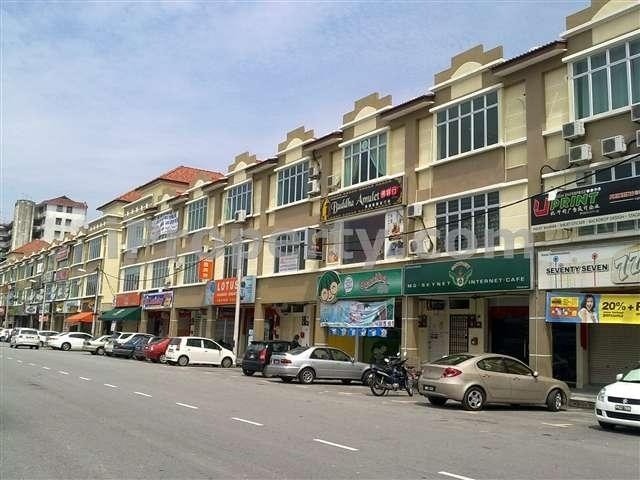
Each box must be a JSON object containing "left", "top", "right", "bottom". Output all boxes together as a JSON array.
[
  {"left": 593, "top": 363, "right": 640, "bottom": 431},
  {"left": 0, "top": 326, "right": 172, "bottom": 367},
  {"left": 263, "top": 341, "right": 375, "bottom": 386},
  {"left": 416, "top": 351, "right": 573, "bottom": 412}
]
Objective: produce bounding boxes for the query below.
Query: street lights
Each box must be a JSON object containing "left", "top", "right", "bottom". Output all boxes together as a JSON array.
[
  {"left": 76, "top": 268, "right": 101, "bottom": 337},
  {"left": 29, "top": 279, "right": 49, "bottom": 331},
  {"left": 210, "top": 238, "right": 244, "bottom": 358}
]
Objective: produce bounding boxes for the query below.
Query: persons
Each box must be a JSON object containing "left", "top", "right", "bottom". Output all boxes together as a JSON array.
[{"left": 577, "top": 295, "right": 598, "bottom": 323}]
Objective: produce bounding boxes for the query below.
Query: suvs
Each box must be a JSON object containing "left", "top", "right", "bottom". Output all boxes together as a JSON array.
[{"left": 240, "top": 340, "right": 306, "bottom": 378}]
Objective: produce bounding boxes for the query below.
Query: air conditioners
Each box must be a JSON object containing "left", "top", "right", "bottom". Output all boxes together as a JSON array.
[
  {"left": 201, "top": 245, "right": 209, "bottom": 254},
  {"left": 409, "top": 240, "right": 422, "bottom": 254},
  {"left": 326, "top": 175, "right": 340, "bottom": 189},
  {"left": 173, "top": 261, "right": 183, "bottom": 270},
  {"left": 307, "top": 167, "right": 320, "bottom": 195},
  {"left": 234, "top": 210, "right": 247, "bottom": 224},
  {"left": 561, "top": 103, "right": 640, "bottom": 165},
  {"left": 406, "top": 202, "right": 422, "bottom": 218}
]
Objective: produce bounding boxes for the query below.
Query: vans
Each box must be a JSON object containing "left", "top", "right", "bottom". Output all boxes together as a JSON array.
[{"left": 165, "top": 334, "right": 236, "bottom": 369}]
